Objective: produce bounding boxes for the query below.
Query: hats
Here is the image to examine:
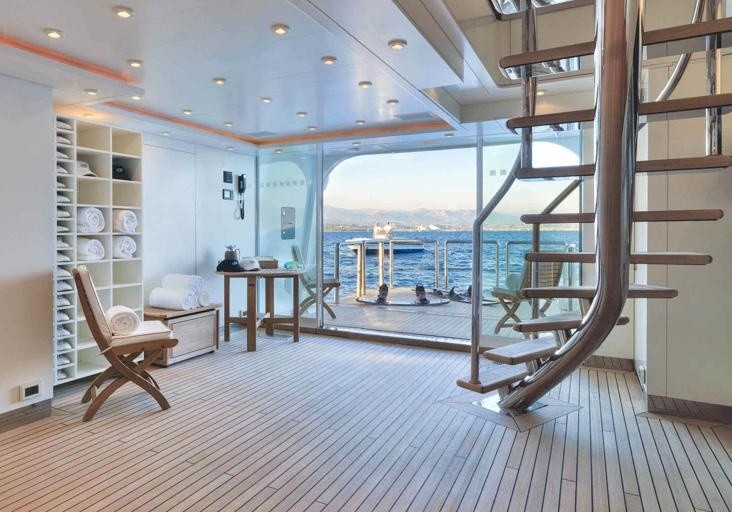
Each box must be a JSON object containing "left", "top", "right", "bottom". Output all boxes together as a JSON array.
[
  {"left": 77, "top": 160, "right": 97, "bottom": 176},
  {"left": 217, "top": 256, "right": 263, "bottom": 272},
  {"left": 113, "top": 165, "right": 131, "bottom": 180}
]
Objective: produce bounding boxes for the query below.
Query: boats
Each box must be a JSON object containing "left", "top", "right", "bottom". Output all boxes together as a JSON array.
[{"left": 345, "top": 220, "right": 425, "bottom": 257}]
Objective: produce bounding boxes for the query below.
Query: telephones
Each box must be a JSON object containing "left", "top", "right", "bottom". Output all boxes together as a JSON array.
[{"left": 238, "top": 173, "right": 246, "bottom": 193}]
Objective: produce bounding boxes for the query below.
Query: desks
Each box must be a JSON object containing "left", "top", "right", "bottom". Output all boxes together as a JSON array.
[{"left": 213, "top": 269, "right": 311, "bottom": 351}]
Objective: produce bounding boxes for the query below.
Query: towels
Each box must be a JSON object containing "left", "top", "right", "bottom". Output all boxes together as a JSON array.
[
  {"left": 78, "top": 236, "right": 105, "bottom": 262},
  {"left": 111, "top": 235, "right": 138, "bottom": 261},
  {"left": 163, "top": 274, "right": 205, "bottom": 296},
  {"left": 198, "top": 292, "right": 211, "bottom": 306},
  {"left": 104, "top": 304, "right": 141, "bottom": 335},
  {"left": 78, "top": 206, "right": 106, "bottom": 233},
  {"left": 149, "top": 287, "right": 196, "bottom": 311},
  {"left": 111, "top": 205, "right": 140, "bottom": 233}
]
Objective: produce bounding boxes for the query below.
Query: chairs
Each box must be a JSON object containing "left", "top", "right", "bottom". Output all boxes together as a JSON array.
[
  {"left": 291, "top": 247, "right": 341, "bottom": 320},
  {"left": 70, "top": 264, "right": 183, "bottom": 422},
  {"left": 493, "top": 250, "right": 570, "bottom": 337}
]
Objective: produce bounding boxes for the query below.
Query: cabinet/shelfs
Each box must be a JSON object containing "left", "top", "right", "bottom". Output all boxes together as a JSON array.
[
  {"left": 54, "top": 115, "right": 147, "bottom": 393},
  {"left": 143, "top": 303, "right": 225, "bottom": 365}
]
